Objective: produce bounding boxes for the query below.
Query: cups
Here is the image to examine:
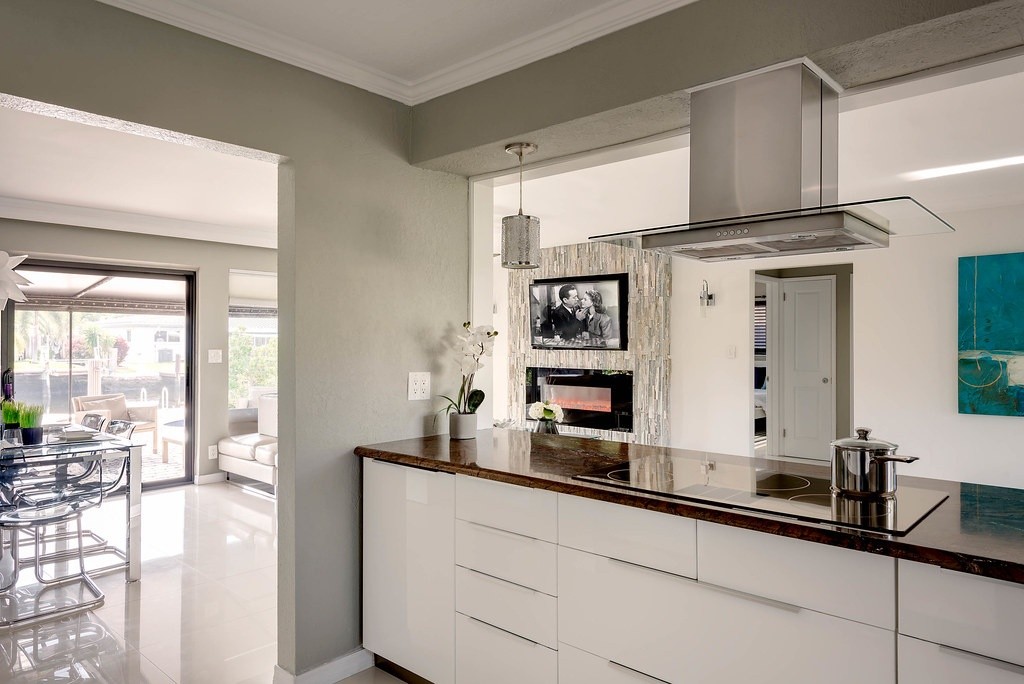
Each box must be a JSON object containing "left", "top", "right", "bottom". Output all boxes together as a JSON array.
[
  {"left": 1, "top": 428, "right": 23, "bottom": 453},
  {"left": 47, "top": 426, "right": 68, "bottom": 446}
]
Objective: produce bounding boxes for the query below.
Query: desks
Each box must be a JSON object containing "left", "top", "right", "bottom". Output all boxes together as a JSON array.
[{"left": 0, "top": 419, "right": 147, "bottom": 582}]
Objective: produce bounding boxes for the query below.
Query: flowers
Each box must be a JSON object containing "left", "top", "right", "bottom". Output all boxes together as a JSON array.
[
  {"left": 437, "top": 319, "right": 498, "bottom": 414},
  {"left": 527, "top": 397, "right": 562, "bottom": 418}
]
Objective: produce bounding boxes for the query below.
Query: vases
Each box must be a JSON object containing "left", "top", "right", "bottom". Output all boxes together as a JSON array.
[
  {"left": 535, "top": 420, "right": 557, "bottom": 435},
  {"left": 449, "top": 415, "right": 477, "bottom": 441}
]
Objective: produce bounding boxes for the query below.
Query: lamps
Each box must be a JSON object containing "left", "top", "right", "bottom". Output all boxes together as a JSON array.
[{"left": 502, "top": 144, "right": 543, "bottom": 271}]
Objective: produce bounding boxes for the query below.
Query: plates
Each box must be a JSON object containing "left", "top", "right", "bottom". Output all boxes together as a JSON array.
[{"left": 63, "top": 433, "right": 93, "bottom": 440}]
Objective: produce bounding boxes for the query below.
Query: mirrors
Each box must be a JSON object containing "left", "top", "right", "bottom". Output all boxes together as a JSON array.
[{"left": 749, "top": 261, "right": 855, "bottom": 506}]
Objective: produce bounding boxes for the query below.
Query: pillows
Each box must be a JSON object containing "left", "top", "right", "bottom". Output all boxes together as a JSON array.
[{"left": 72, "top": 394, "right": 129, "bottom": 421}]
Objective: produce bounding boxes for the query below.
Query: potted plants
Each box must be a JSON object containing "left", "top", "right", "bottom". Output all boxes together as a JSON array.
[
  {"left": 19, "top": 406, "right": 44, "bottom": 446},
  {"left": 1, "top": 403, "right": 19, "bottom": 438}
]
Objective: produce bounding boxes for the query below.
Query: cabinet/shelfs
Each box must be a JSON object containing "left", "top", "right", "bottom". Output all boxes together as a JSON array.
[{"left": 362, "top": 459, "right": 1024, "bottom": 684}]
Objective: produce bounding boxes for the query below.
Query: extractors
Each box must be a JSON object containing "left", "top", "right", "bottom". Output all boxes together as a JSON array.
[{"left": 632, "top": 212, "right": 893, "bottom": 263}]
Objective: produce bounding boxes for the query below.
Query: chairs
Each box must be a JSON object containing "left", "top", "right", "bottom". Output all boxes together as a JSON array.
[
  {"left": 16, "top": 420, "right": 137, "bottom": 585},
  {"left": 0, "top": 413, "right": 106, "bottom": 542},
  {"left": 0, "top": 454, "right": 103, "bottom": 631}
]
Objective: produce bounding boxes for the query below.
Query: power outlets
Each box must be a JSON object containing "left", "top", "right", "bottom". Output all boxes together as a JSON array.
[
  {"left": 409, "top": 372, "right": 432, "bottom": 400},
  {"left": 208, "top": 446, "right": 218, "bottom": 460}
]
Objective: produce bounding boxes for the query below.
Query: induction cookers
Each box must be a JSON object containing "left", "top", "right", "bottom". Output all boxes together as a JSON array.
[{"left": 572, "top": 452, "right": 948, "bottom": 538}]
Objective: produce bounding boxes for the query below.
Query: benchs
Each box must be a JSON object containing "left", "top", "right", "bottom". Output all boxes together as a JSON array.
[{"left": 71, "top": 406, "right": 158, "bottom": 455}]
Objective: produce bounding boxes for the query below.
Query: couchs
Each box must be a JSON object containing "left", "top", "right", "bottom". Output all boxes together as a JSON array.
[{"left": 219, "top": 395, "right": 277, "bottom": 500}]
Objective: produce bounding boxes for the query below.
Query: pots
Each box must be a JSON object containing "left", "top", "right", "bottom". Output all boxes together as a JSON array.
[{"left": 829, "top": 426, "right": 918, "bottom": 497}]
